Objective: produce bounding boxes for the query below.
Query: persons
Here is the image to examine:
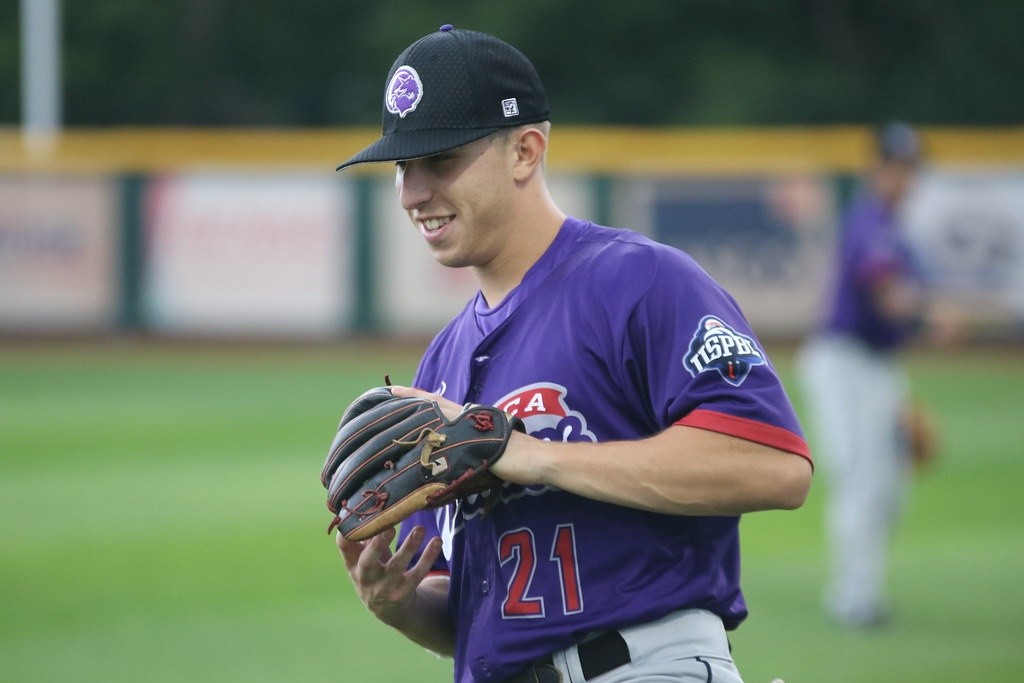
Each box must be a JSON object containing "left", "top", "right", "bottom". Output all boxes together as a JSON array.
[
  {"left": 321, "top": 23, "right": 816, "bottom": 681},
  {"left": 800, "top": 121, "right": 957, "bottom": 625}
]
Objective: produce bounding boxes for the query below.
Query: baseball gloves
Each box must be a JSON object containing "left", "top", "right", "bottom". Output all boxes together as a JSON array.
[{"left": 320, "top": 381, "right": 525, "bottom": 548}]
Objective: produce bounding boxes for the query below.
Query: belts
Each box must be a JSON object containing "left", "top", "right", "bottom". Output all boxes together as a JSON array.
[{"left": 505, "top": 624, "right": 634, "bottom": 683}]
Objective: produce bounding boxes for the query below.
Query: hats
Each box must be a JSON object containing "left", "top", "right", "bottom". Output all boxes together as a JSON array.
[
  {"left": 334, "top": 23, "right": 551, "bottom": 174},
  {"left": 870, "top": 119, "right": 942, "bottom": 178}
]
